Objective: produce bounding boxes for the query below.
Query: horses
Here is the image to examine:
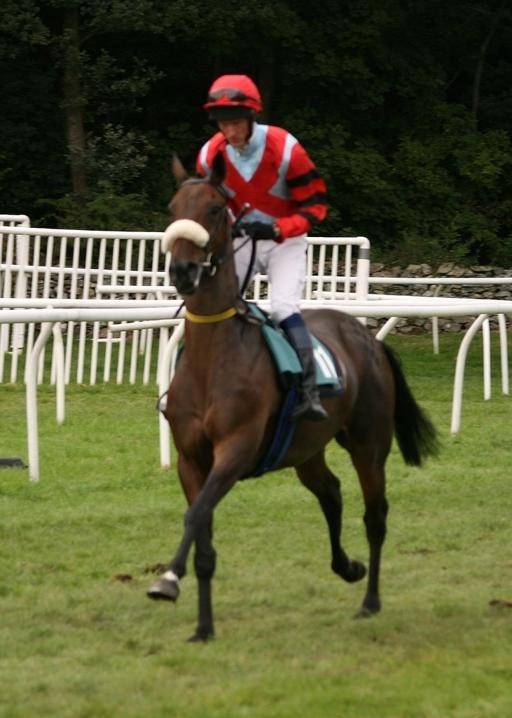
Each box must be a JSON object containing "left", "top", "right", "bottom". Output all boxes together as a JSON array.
[{"left": 144, "top": 151, "right": 442, "bottom": 646}]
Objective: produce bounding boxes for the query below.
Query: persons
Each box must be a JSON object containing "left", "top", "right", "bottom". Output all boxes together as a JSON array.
[{"left": 189, "top": 70, "right": 332, "bottom": 427}]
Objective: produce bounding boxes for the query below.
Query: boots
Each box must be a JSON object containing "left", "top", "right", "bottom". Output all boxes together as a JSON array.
[{"left": 282, "top": 315, "right": 332, "bottom": 424}]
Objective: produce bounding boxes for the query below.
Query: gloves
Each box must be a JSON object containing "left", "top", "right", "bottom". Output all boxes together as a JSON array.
[{"left": 246, "top": 219, "right": 276, "bottom": 240}]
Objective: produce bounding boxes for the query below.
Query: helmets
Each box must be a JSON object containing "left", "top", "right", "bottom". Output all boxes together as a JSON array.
[{"left": 202, "top": 71, "right": 264, "bottom": 128}]
